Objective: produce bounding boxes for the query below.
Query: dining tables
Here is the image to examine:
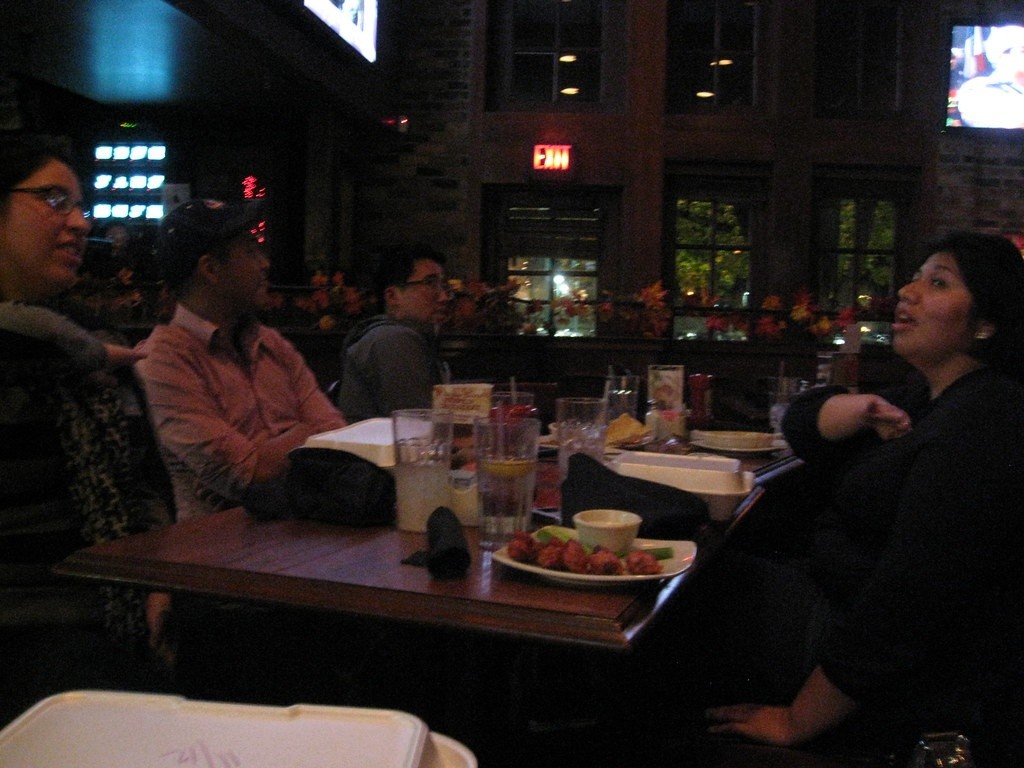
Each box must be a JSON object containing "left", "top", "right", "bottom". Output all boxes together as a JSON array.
[{"left": 54, "top": 452, "right": 802, "bottom": 650}]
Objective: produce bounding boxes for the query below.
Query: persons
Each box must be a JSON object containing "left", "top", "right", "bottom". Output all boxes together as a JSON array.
[
  {"left": 334, "top": 240, "right": 449, "bottom": 422},
  {"left": 0, "top": 128, "right": 207, "bottom": 728},
  {"left": 681, "top": 228, "right": 1024, "bottom": 765},
  {"left": 88, "top": 216, "right": 146, "bottom": 310},
  {"left": 0, "top": 301, "right": 150, "bottom": 383},
  {"left": 132, "top": 200, "right": 360, "bottom": 662}
]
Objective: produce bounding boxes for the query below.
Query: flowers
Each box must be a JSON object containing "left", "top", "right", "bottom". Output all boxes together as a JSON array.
[{"left": 48, "top": 266, "right": 900, "bottom": 340}]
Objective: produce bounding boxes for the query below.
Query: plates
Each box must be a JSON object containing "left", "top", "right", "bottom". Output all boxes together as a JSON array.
[
  {"left": 535, "top": 432, "right": 656, "bottom": 452},
  {"left": 690, "top": 432, "right": 788, "bottom": 452},
  {"left": 490, "top": 522, "right": 699, "bottom": 587}
]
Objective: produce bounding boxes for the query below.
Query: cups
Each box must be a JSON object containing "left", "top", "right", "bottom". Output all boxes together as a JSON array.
[
  {"left": 768, "top": 375, "right": 799, "bottom": 431},
  {"left": 604, "top": 376, "right": 641, "bottom": 420},
  {"left": 555, "top": 396, "right": 608, "bottom": 481},
  {"left": 491, "top": 391, "right": 535, "bottom": 416},
  {"left": 474, "top": 416, "right": 540, "bottom": 552},
  {"left": 392, "top": 409, "right": 454, "bottom": 534}
]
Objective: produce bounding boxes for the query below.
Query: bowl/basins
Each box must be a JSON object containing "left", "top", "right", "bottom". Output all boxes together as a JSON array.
[
  {"left": 607, "top": 451, "right": 755, "bottom": 522},
  {"left": 572, "top": 510, "right": 642, "bottom": 559}
]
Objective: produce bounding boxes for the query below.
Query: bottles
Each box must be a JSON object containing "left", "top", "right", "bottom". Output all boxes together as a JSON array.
[{"left": 645, "top": 396, "right": 663, "bottom": 432}]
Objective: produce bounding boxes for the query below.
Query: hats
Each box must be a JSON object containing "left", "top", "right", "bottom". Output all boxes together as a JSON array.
[{"left": 157, "top": 197, "right": 265, "bottom": 259}]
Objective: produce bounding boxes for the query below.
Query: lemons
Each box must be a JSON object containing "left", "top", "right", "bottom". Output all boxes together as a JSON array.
[{"left": 484, "top": 458, "right": 536, "bottom": 477}]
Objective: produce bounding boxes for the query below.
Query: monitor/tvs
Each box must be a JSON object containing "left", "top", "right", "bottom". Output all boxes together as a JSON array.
[
  {"left": 296, "top": 1, "right": 385, "bottom": 70},
  {"left": 938, "top": 16, "right": 1024, "bottom": 144}
]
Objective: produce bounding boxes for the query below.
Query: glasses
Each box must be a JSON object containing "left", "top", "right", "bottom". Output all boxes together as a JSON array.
[
  {"left": 9, "top": 184, "right": 97, "bottom": 223},
  {"left": 399, "top": 273, "right": 450, "bottom": 292}
]
